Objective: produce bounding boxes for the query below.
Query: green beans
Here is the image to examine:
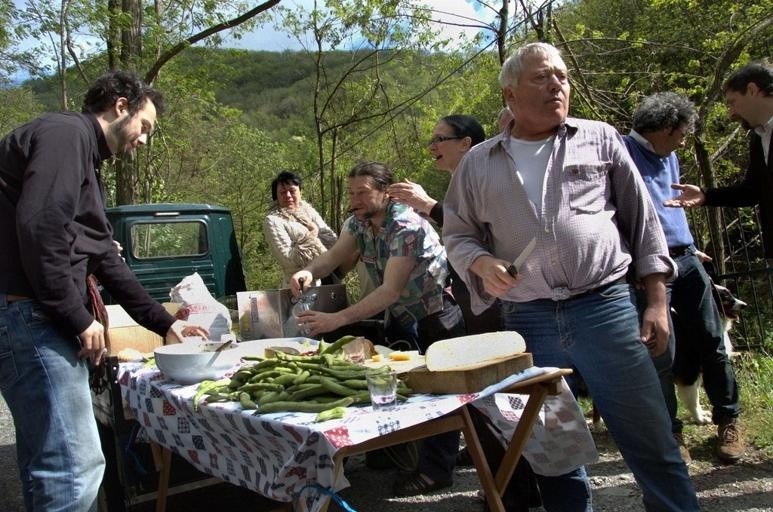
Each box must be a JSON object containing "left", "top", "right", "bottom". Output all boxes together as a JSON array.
[{"left": 193, "top": 336, "right": 413, "bottom": 423}]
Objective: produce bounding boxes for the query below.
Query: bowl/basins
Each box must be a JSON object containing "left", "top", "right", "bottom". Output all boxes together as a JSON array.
[{"left": 154, "top": 342, "right": 238, "bottom": 386}]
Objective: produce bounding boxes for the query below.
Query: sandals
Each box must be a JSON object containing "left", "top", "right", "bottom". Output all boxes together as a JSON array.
[{"left": 393, "top": 468, "right": 453, "bottom": 497}]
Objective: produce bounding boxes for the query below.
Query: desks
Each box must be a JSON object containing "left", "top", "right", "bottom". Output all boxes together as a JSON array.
[{"left": 117, "top": 337, "right": 572, "bottom": 511}]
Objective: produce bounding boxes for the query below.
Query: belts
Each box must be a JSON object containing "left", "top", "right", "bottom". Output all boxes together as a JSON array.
[{"left": 668, "top": 246, "right": 692, "bottom": 256}]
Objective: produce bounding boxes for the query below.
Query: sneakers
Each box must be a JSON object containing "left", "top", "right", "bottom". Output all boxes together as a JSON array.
[
  {"left": 673, "top": 431, "right": 691, "bottom": 466},
  {"left": 717, "top": 422, "right": 744, "bottom": 461}
]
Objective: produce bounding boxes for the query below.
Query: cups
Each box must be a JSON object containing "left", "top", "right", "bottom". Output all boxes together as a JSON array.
[
  {"left": 366, "top": 371, "right": 396, "bottom": 414},
  {"left": 291, "top": 301, "right": 316, "bottom": 337}
]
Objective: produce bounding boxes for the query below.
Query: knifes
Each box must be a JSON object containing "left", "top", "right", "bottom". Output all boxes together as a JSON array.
[{"left": 506, "top": 235, "right": 538, "bottom": 277}]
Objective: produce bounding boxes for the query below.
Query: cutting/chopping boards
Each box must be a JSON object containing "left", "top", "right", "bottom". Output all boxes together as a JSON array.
[{"left": 405, "top": 353, "right": 533, "bottom": 395}]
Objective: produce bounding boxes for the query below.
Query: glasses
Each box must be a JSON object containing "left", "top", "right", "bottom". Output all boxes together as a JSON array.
[{"left": 430, "top": 136, "right": 463, "bottom": 144}]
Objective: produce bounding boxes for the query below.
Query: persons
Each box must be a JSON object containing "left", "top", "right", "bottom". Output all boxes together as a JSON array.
[
  {"left": 662, "top": 65, "right": 773, "bottom": 262},
  {"left": 440, "top": 41, "right": 702, "bottom": 512},
  {"left": 620, "top": 91, "right": 746, "bottom": 466},
  {"left": 385, "top": 114, "right": 542, "bottom": 511},
  {"left": 263, "top": 171, "right": 339, "bottom": 290},
  {"left": 289, "top": 161, "right": 465, "bottom": 495},
  {"left": 0, "top": 71, "right": 212, "bottom": 512}
]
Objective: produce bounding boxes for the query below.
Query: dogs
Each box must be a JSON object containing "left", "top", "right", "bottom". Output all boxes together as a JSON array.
[{"left": 675, "top": 282, "right": 748, "bottom": 425}]
